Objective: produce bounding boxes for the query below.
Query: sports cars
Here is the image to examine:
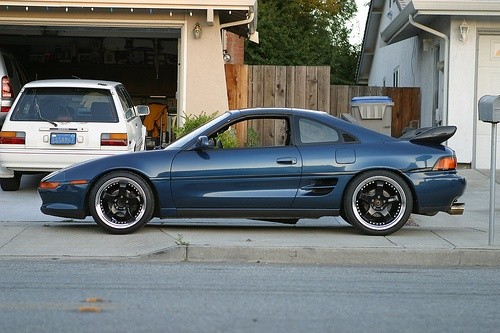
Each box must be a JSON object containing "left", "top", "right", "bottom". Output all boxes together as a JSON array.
[{"left": 36, "top": 108, "right": 468, "bottom": 234}]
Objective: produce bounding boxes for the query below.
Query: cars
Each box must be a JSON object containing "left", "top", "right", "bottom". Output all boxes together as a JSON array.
[{"left": 0, "top": 79, "right": 150, "bottom": 192}]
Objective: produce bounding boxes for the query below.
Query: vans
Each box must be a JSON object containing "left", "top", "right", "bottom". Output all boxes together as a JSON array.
[{"left": 0, "top": 49, "right": 28, "bottom": 128}]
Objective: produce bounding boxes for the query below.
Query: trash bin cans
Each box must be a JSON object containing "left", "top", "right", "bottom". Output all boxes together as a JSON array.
[{"left": 349, "top": 95, "right": 394, "bottom": 138}]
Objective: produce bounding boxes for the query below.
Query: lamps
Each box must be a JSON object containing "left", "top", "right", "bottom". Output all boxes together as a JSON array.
[
  {"left": 193, "top": 22, "right": 203, "bottom": 38},
  {"left": 459, "top": 18, "right": 470, "bottom": 42}
]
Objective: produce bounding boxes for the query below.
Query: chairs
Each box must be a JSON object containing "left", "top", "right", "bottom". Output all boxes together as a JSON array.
[{"left": 34, "top": 101, "right": 115, "bottom": 122}]
